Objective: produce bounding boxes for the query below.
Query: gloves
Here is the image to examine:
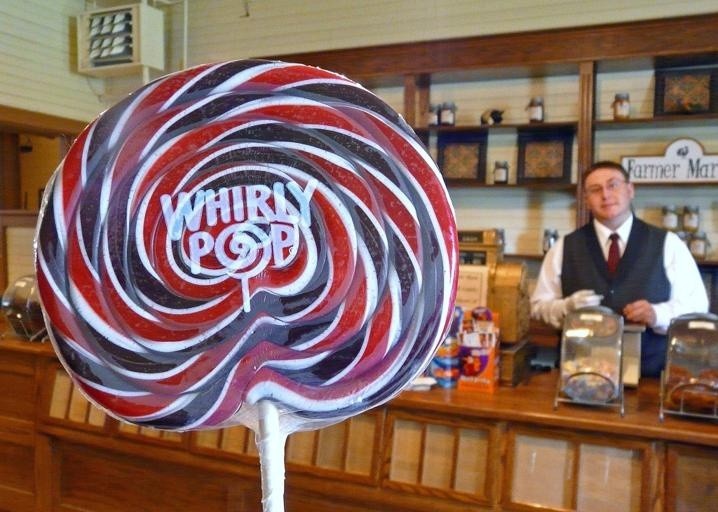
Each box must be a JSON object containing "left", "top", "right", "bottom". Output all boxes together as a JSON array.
[{"left": 560, "top": 290, "right": 607, "bottom": 315}]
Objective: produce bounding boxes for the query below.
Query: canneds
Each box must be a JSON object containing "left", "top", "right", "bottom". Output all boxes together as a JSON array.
[
  {"left": 495, "top": 160, "right": 509, "bottom": 181},
  {"left": 662, "top": 205, "right": 708, "bottom": 260},
  {"left": 613, "top": 93, "right": 631, "bottom": 118},
  {"left": 527, "top": 95, "right": 544, "bottom": 122},
  {"left": 439, "top": 103, "right": 455, "bottom": 125},
  {"left": 428, "top": 103, "right": 440, "bottom": 125},
  {"left": 541, "top": 229, "right": 557, "bottom": 252}
]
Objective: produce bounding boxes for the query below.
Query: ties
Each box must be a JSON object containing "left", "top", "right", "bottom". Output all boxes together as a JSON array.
[
  {"left": 583, "top": 176, "right": 628, "bottom": 191},
  {"left": 603, "top": 229, "right": 624, "bottom": 275}
]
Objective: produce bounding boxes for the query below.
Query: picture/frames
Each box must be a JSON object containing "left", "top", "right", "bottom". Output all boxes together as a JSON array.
[
  {"left": 517, "top": 136, "right": 571, "bottom": 184},
  {"left": 437, "top": 136, "right": 488, "bottom": 185},
  {"left": 653, "top": 67, "right": 718, "bottom": 117}
]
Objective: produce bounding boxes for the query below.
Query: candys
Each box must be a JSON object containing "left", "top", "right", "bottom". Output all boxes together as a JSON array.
[{"left": 34, "top": 58, "right": 460, "bottom": 512}]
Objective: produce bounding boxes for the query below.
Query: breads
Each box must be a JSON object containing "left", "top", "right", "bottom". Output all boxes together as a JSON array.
[
  {"left": 571, "top": 313, "right": 618, "bottom": 336},
  {"left": 562, "top": 356, "right": 615, "bottom": 384},
  {"left": 4, "top": 298, "right": 43, "bottom": 334},
  {"left": 664, "top": 365, "right": 717, "bottom": 410}
]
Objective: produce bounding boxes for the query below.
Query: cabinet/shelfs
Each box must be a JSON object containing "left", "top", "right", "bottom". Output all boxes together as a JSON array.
[
  {"left": 296, "top": 14, "right": 718, "bottom": 351},
  {"left": 0, "top": 105, "right": 93, "bottom": 318}
]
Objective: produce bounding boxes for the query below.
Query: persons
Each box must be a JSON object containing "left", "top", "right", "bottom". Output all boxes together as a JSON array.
[{"left": 530, "top": 161, "right": 709, "bottom": 378}]
